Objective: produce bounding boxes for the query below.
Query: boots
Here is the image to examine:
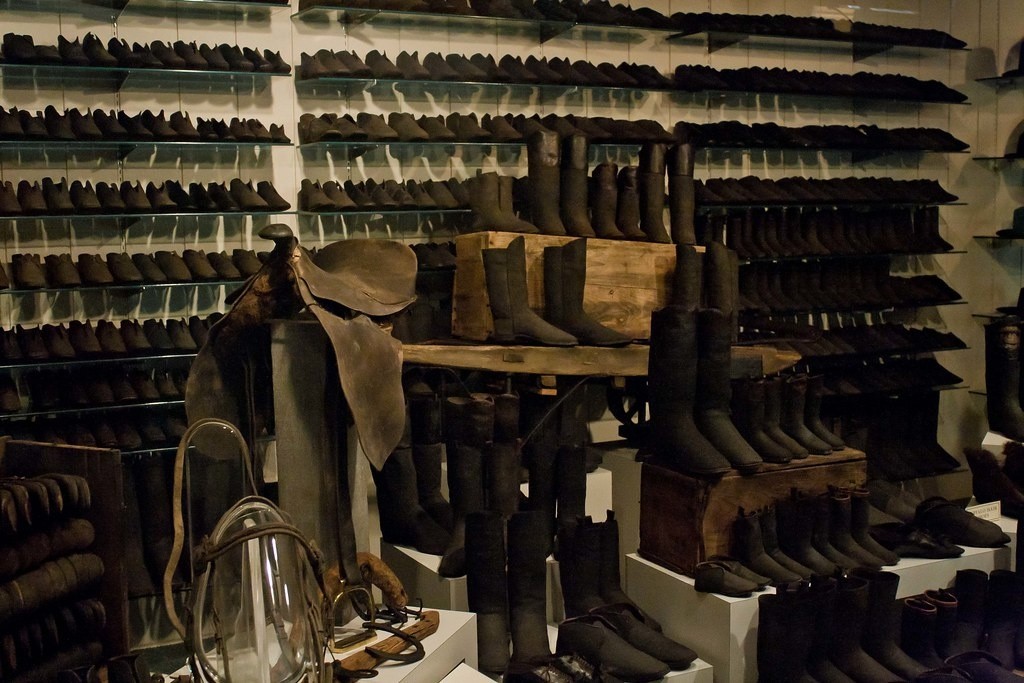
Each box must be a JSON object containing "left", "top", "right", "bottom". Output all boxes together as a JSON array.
[{"left": 391, "top": 131, "right": 1024, "bottom": 683}]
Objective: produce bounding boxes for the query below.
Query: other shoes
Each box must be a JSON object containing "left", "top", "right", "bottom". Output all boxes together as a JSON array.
[
  {"left": 0, "top": 174, "right": 392, "bottom": 683},
  {"left": 0, "top": 0, "right": 969, "bottom": 152}
]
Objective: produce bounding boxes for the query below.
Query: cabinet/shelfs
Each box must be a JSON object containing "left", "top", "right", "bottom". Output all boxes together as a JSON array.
[{"left": 0, "top": 0, "right": 1024, "bottom": 683}]
